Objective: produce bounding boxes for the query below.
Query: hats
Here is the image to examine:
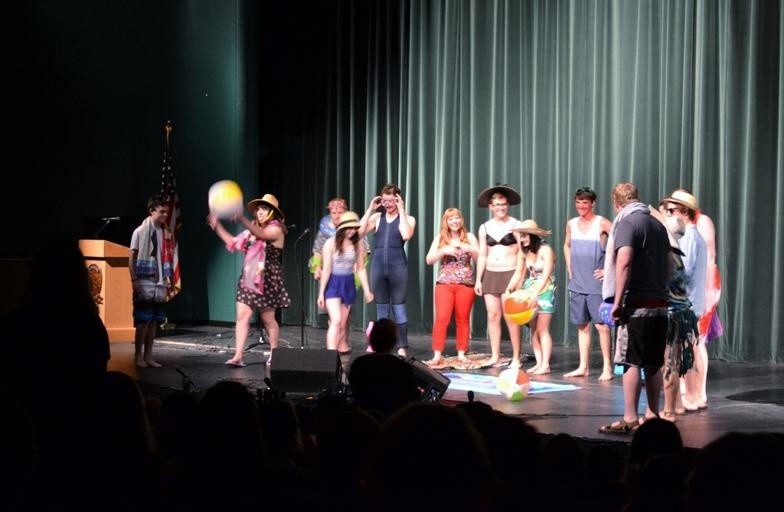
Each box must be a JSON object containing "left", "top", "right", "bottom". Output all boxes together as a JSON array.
[
  {"left": 478, "top": 182, "right": 521, "bottom": 208},
  {"left": 648, "top": 205, "right": 685, "bottom": 257},
  {"left": 336, "top": 212, "right": 361, "bottom": 233},
  {"left": 663, "top": 191, "right": 697, "bottom": 210},
  {"left": 247, "top": 193, "right": 286, "bottom": 222},
  {"left": 509, "top": 219, "right": 552, "bottom": 237}
]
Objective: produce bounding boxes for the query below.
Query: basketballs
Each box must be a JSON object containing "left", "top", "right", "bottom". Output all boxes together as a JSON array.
[
  {"left": 502, "top": 292, "right": 537, "bottom": 325},
  {"left": 208, "top": 180, "right": 242, "bottom": 220},
  {"left": 497, "top": 368, "right": 530, "bottom": 402}
]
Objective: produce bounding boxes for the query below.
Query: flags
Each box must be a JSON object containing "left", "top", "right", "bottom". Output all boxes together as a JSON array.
[{"left": 161, "top": 122, "right": 182, "bottom": 238}]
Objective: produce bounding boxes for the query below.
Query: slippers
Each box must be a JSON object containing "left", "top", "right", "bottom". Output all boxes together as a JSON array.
[{"left": 225, "top": 358, "right": 246, "bottom": 368}]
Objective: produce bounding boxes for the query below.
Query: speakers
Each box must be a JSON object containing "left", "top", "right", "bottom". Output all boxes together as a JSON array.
[{"left": 270, "top": 348, "right": 343, "bottom": 393}]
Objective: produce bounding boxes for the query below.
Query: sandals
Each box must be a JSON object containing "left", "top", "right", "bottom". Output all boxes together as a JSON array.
[{"left": 599, "top": 418, "right": 639, "bottom": 435}]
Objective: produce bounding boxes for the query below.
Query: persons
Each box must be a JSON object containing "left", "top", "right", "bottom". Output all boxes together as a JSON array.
[
  {"left": 207, "top": 190, "right": 292, "bottom": 366},
  {"left": 1, "top": 238, "right": 782, "bottom": 512},
  {"left": 126, "top": 198, "right": 181, "bottom": 372},
  {"left": 311, "top": 182, "right": 721, "bottom": 432}
]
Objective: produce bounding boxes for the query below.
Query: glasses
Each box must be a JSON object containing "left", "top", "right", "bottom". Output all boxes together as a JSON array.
[{"left": 667, "top": 207, "right": 681, "bottom": 215}]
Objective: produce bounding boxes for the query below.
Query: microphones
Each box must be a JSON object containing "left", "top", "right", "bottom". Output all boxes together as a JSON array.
[
  {"left": 173, "top": 364, "right": 194, "bottom": 384},
  {"left": 299, "top": 228, "right": 309, "bottom": 240},
  {"left": 104, "top": 217, "right": 120, "bottom": 221}
]
